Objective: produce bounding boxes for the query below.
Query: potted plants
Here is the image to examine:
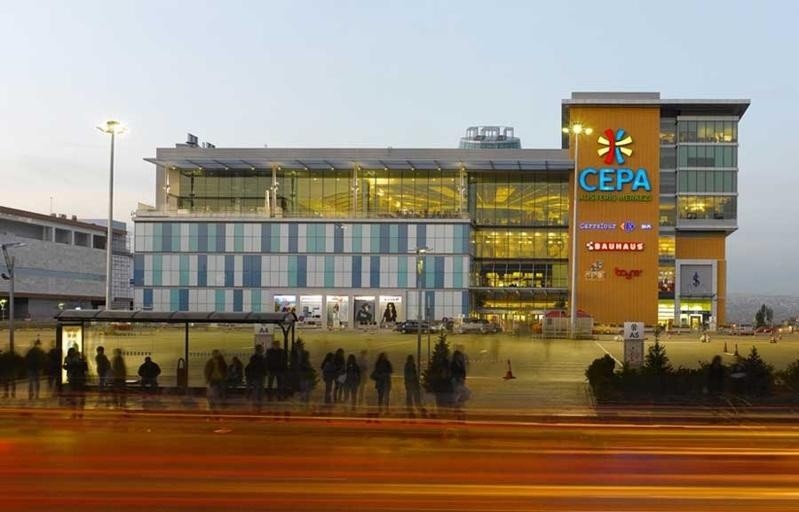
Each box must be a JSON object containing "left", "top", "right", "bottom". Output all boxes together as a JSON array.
[{"left": 582, "top": 353, "right": 617, "bottom": 398}]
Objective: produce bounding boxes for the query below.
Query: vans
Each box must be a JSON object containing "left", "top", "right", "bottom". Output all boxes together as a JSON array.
[
  {"left": 753, "top": 326, "right": 773, "bottom": 336},
  {"left": 728, "top": 323, "right": 753, "bottom": 335}
]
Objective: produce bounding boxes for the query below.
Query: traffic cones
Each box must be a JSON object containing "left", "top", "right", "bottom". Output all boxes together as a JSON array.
[
  {"left": 502, "top": 359, "right": 516, "bottom": 379},
  {"left": 733, "top": 344, "right": 740, "bottom": 356},
  {"left": 723, "top": 342, "right": 729, "bottom": 352}
]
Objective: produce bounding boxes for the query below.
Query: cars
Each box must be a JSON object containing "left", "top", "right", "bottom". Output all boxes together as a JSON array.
[
  {"left": 593, "top": 320, "right": 624, "bottom": 335},
  {"left": 393, "top": 320, "right": 438, "bottom": 334},
  {"left": 455, "top": 317, "right": 498, "bottom": 335}
]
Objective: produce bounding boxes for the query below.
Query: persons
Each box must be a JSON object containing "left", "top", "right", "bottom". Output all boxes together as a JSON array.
[
  {"left": 380, "top": 302, "right": 397, "bottom": 327},
  {"left": 332, "top": 303, "right": 340, "bottom": 321},
  {"left": 282, "top": 304, "right": 319, "bottom": 319},
  {"left": 203, "top": 338, "right": 467, "bottom": 412},
  {"left": 703, "top": 356, "right": 724, "bottom": 406},
  {"left": 0, "top": 339, "right": 162, "bottom": 406},
  {"left": 356, "top": 302, "right": 372, "bottom": 322}
]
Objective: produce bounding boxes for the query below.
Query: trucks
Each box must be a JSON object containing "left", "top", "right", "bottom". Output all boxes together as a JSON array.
[{"left": 531, "top": 309, "right": 593, "bottom": 334}]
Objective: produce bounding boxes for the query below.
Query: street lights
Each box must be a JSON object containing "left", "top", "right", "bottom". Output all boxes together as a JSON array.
[
  {"left": 58, "top": 303, "right": 67, "bottom": 310},
  {"left": 561, "top": 120, "right": 594, "bottom": 339},
  {"left": 0, "top": 240, "right": 29, "bottom": 356},
  {"left": 97, "top": 120, "right": 126, "bottom": 311},
  {"left": 0, "top": 298, "right": 9, "bottom": 320},
  {"left": 414, "top": 247, "right": 432, "bottom": 381}
]
